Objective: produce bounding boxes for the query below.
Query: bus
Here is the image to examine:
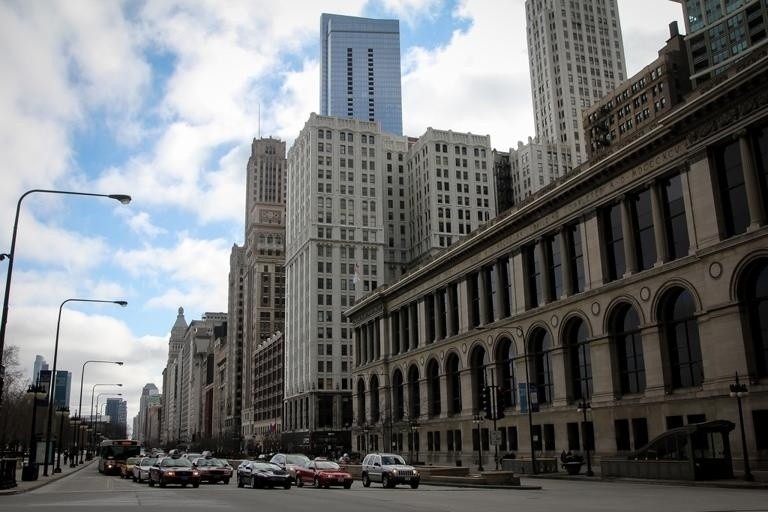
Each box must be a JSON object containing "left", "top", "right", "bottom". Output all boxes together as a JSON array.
[{"left": 94, "top": 439, "right": 141, "bottom": 475}]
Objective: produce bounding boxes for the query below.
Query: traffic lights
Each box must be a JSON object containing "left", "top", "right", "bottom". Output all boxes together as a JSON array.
[
  {"left": 482, "top": 386, "right": 491, "bottom": 419},
  {"left": 496, "top": 389, "right": 506, "bottom": 419}
]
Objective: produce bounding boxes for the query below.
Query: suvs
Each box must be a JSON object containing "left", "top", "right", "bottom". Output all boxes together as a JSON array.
[{"left": 362, "top": 452, "right": 420, "bottom": 490}]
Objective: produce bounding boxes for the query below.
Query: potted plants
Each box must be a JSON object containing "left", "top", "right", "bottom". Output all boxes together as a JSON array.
[{"left": 558, "top": 450, "right": 587, "bottom": 478}]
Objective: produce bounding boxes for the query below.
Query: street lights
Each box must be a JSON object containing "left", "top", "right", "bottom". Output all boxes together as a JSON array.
[
  {"left": 472, "top": 410, "right": 485, "bottom": 472},
  {"left": 68, "top": 409, "right": 105, "bottom": 468},
  {"left": 357, "top": 372, "right": 393, "bottom": 451},
  {"left": 93, "top": 393, "right": 123, "bottom": 453},
  {"left": 408, "top": 419, "right": 420, "bottom": 465},
  {"left": 362, "top": 422, "right": 370, "bottom": 456},
  {"left": 75, "top": 360, "right": 126, "bottom": 467},
  {"left": 474, "top": 326, "right": 537, "bottom": 475},
  {"left": 87, "top": 383, "right": 124, "bottom": 459},
  {"left": 729, "top": 369, "right": 754, "bottom": 482},
  {"left": 40, "top": 296, "right": 129, "bottom": 480},
  {"left": 21, "top": 372, "right": 48, "bottom": 482},
  {"left": 53, "top": 402, "right": 70, "bottom": 473},
  {"left": 576, "top": 396, "right": 592, "bottom": 477},
  {"left": 0, "top": 189, "right": 133, "bottom": 371}
]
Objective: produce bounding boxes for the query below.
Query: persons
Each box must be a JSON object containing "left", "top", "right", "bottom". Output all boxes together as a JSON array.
[
  {"left": 561, "top": 448, "right": 572, "bottom": 469},
  {"left": 64, "top": 448, "right": 72, "bottom": 465}
]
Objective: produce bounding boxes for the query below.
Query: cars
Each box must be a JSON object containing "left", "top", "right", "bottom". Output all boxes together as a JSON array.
[
  {"left": 120, "top": 448, "right": 234, "bottom": 488},
  {"left": 270, "top": 453, "right": 312, "bottom": 482},
  {"left": 293, "top": 456, "right": 353, "bottom": 491},
  {"left": 236, "top": 459, "right": 292, "bottom": 489}
]
